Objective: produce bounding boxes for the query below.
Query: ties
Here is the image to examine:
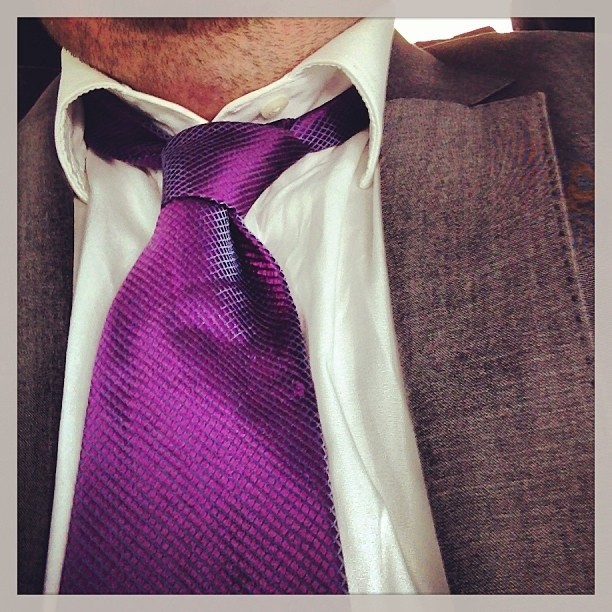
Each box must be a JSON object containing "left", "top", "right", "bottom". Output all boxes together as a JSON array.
[{"left": 59, "top": 84, "right": 369, "bottom": 595}]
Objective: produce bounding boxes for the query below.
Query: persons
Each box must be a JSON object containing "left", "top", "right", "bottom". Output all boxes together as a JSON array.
[{"left": 18, "top": 18, "right": 594, "bottom": 595}]
